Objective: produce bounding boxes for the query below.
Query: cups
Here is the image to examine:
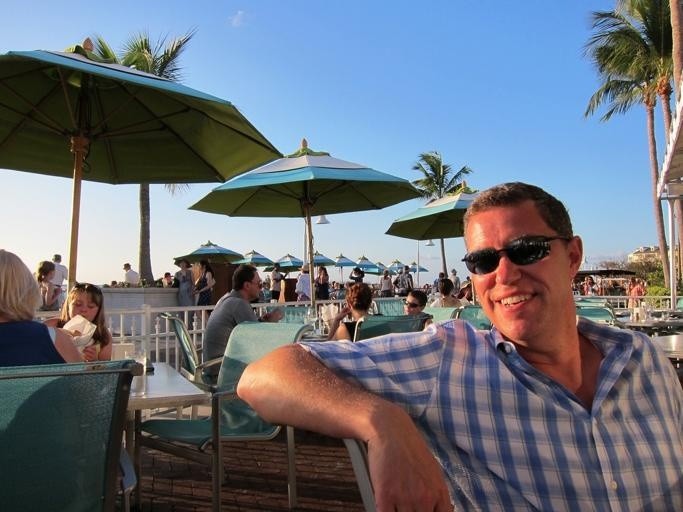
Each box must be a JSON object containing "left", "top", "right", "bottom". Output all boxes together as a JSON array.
[
  {"left": 125, "top": 349, "right": 146, "bottom": 397},
  {"left": 307, "top": 306, "right": 318, "bottom": 335}
]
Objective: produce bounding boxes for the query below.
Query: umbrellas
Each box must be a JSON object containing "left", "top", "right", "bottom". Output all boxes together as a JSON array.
[
  {"left": 409, "top": 261, "right": 428, "bottom": 272},
  {"left": 2, "top": 38, "right": 282, "bottom": 285},
  {"left": 387, "top": 259, "right": 410, "bottom": 274},
  {"left": 312, "top": 250, "right": 335, "bottom": 266},
  {"left": 188, "top": 139, "right": 422, "bottom": 301},
  {"left": 362, "top": 262, "right": 395, "bottom": 274},
  {"left": 174, "top": 240, "right": 245, "bottom": 266},
  {"left": 232, "top": 249, "right": 275, "bottom": 266},
  {"left": 355, "top": 255, "right": 378, "bottom": 268},
  {"left": 336, "top": 253, "right": 358, "bottom": 267},
  {"left": 275, "top": 253, "right": 304, "bottom": 272},
  {"left": 384, "top": 180, "right": 480, "bottom": 240}
]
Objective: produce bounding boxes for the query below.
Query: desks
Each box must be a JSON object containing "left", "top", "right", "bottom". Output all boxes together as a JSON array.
[
  {"left": 109, "top": 359, "right": 210, "bottom": 412},
  {"left": 653, "top": 307, "right": 683, "bottom": 316},
  {"left": 624, "top": 316, "right": 680, "bottom": 330},
  {"left": 651, "top": 330, "right": 683, "bottom": 364}
]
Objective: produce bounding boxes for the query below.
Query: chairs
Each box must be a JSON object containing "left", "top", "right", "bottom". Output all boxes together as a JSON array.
[
  {"left": 579, "top": 298, "right": 607, "bottom": 307},
  {"left": 265, "top": 305, "right": 317, "bottom": 328},
  {"left": 575, "top": 307, "right": 612, "bottom": 325},
  {"left": 456, "top": 305, "right": 491, "bottom": 331},
  {"left": 420, "top": 302, "right": 459, "bottom": 325},
  {"left": 349, "top": 313, "right": 433, "bottom": 342},
  {"left": 341, "top": 433, "right": 378, "bottom": 507},
  {"left": 0, "top": 359, "right": 143, "bottom": 512},
  {"left": 368, "top": 297, "right": 409, "bottom": 316},
  {"left": 158, "top": 311, "right": 225, "bottom": 393},
  {"left": 137, "top": 319, "right": 317, "bottom": 508}
]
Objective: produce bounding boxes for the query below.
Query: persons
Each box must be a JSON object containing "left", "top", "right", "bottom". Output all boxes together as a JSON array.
[
  {"left": 236, "top": 182, "right": 683, "bottom": 511},
  {"left": 335, "top": 283, "right": 383, "bottom": 343},
  {"left": 571, "top": 274, "right": 649, "bottom": 299},
  {"left": 294, "top": 263, "right": 311, "bottom": 302},
  {"left": 35, "top": 261, "right": 61, "bottom": 310},
  {"left": 313, "top": 267, "right": 330, "bottom": 300},
  {"left": 0, "top": 249, "right": 84, "bottom": 366},
  {"left": 460, "top": 275, "right": 474, "bottom": 304},
  {"left": 203, "top": 264, "right": 284, "bottom": 386},
  {"left": 43, "top": 283, "right": 112, "bottom": 360},
  {"left": 434, "top": 279, "right": 462, "bottom": 309},
  {"left": 349, "top": 267, "right": 364, "bottom": 284},
  {"left": 48, "top": 254, "right": 67, "bottom": 288},
  {"left": 124, "top": 263, "right": 139, "bottom": 284},
  {"left": 174, "top": 257, "right": 195, "bottom": 305},
  {"left": 270, "top": 263, "right": 289, "bottom": 299},
  {"left": 402, "top": 291, "right": 434, "bottom": 329},
  {"left": 194, "top": 261, "right": 214, "bottom": 305},
  {"left": 434, "top": 272, "right": 444, "bottom": 287},
  {"left": 393, "top": 265, "right": 413, "bottom": 297},
  {"left": 330, "top": 281, "right": 346, "bottom": 299},
  {"left": 379, "top": 271, "right": 391, "bottom": 297},
  {"left": 162, "top": 272, "right": 174, "bottom": 288},
  {"left": 449, "top": 269, "right": 460, "bottom": 296}
]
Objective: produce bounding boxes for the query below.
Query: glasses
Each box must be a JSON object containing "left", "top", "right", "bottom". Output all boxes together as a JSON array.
[
  {"left": 403, "top": 298, "right": 422, "bottom": 309},
  {"left": 249, "top": 281, "right": 262, "bottom": 287},
  {"left": 459, "top": 234, "right": 571, "bottom": 276}
]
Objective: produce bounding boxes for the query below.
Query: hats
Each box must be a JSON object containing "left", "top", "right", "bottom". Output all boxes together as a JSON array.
[
  {"left": 403, "top": 266, "right": 411, "bottom": 270},
  {"left": 298, "top": 264, "right": 309, "bottom": 272},
  {"left": 398, "top": 269, "right": 403, "bottom": 274}
]
